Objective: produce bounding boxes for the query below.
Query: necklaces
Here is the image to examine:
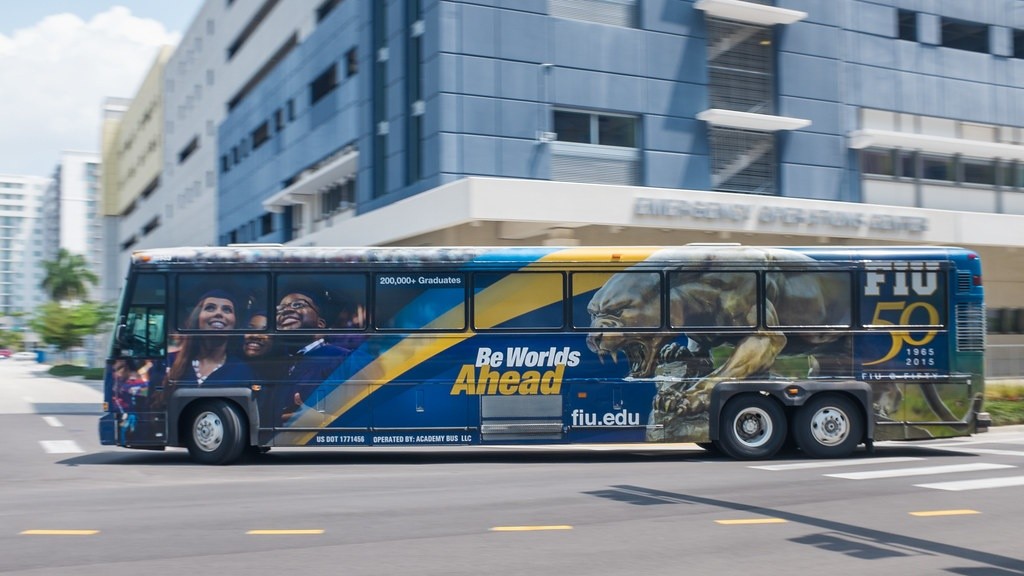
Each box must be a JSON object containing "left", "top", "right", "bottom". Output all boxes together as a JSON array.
[{"left": 191, "top": 360, "right": 222, "bottom": 385}]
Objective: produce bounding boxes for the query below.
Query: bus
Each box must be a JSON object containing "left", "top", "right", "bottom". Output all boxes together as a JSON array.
[{"left": 97, "top": 242, "right": 993, "bottom": 461}]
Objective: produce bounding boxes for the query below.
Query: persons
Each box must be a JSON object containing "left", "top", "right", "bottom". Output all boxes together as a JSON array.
[{"left": 166, "top": 288, "right": 351, "bottom": 445}]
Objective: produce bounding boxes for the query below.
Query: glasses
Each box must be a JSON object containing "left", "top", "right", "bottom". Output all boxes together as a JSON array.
[{"left": 274, "top": 298, "right": 322, "bottom": 318}]
